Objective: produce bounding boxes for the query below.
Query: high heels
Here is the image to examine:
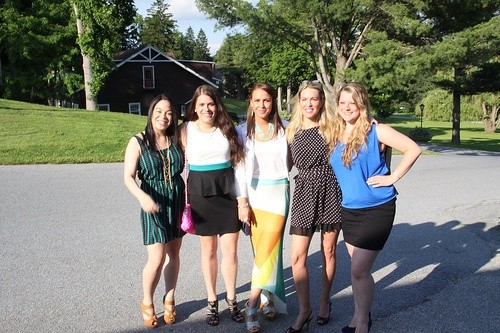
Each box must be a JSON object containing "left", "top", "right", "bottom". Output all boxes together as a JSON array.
[
  {"left": 225, "top": 295, "right": 244, "bottom": 323},
  {"left": 316, "top": 301, "right": 332, "bottom": 325},
  {"left": 141, "top": 301, "right": 157, "bottom": 328},
  {"left": 245, "top": 300, "right": 261, "bottom": 333},
  {"left": 285, "top": 310, "right": 314, "bottom": 333},
  {"left": 342, "top": 320, "right": 371, "bottom": 333},
  {"left": 261, "top": 292, "right": 275, "bottom": 320},
  {"left": 207, "top": 295, "right": 220, "bottom": 326},
  {"left": 163, "top": 294, "right": 177, "bottom": 324}
]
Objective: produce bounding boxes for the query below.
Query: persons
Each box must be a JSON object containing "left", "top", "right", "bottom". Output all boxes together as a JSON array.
[
  {"left": 235, "top": 83, "right": 291, "bottom": 333},
  {"left": 123, "top": 96, "right": 185, "bottom": 328},
  {"left": 329, "top": 83, "right": 422, "bottom": 333},
  {"left": 285, "top": 83, "right": 378, "bottom": 333},
  {"left": 178, "top": 84, "right": 245, "bottom": 326}
]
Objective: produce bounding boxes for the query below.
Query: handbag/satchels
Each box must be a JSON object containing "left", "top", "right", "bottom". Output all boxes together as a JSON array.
[{"left": 181, "top": 203, "right": 194, "bottom": 234}]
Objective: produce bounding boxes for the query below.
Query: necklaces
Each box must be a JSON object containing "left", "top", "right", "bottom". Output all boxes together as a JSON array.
[{"left": 158, "top": 136, "right": 173, "bottom": 190}]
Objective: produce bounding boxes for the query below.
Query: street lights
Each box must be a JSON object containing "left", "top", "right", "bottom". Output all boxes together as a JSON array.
[{"left": 419, "top": 103, "right": 425, "bottom": 128}]
